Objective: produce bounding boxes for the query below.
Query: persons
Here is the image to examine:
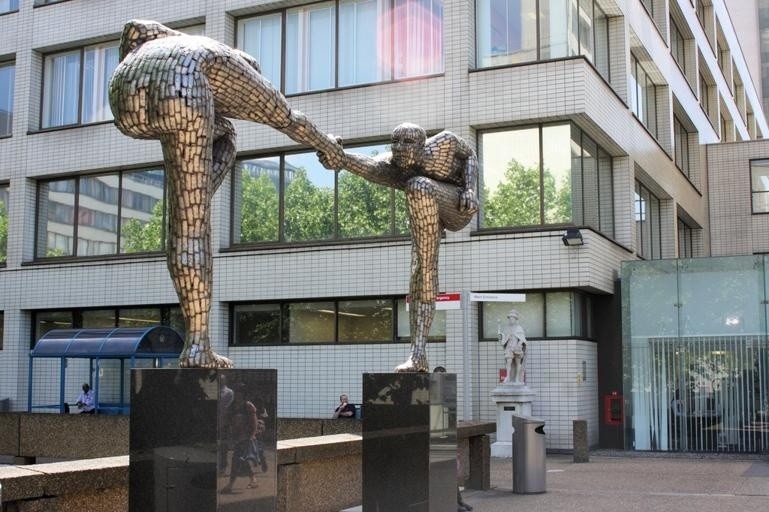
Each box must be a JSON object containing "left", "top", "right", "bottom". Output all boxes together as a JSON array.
[
  {"left": 312, "top": 121, "right": 481, "bottom": 376},
  {"left": 332, "top": 395, "right": 357, "bottom": 420},
  {"left": 495, "top": 309, "right": 530, "bottom": 383},
  {"left": 108, "top": 13, "right": 347, "bottom": 368},
  {"left": 217, "top": 373, "right": 234, "bottom": 480},
  {"left": 434, "top": 366, "right": 447, "bottom": 371},
  {"left": 74, "top": 382, "right": 102, "bottom": 415},
  {"left": 218, "top": 382, "right": 263, "bottom": 495}
]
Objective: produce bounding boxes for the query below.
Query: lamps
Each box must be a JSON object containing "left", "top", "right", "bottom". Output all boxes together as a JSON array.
[{"left": 561, "top": 229, "right": 584, "bottom": 246}]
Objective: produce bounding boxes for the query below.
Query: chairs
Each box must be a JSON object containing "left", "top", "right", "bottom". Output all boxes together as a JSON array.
[{"left": 343, "top": 402, "right": 361, "bottom": 413}]
